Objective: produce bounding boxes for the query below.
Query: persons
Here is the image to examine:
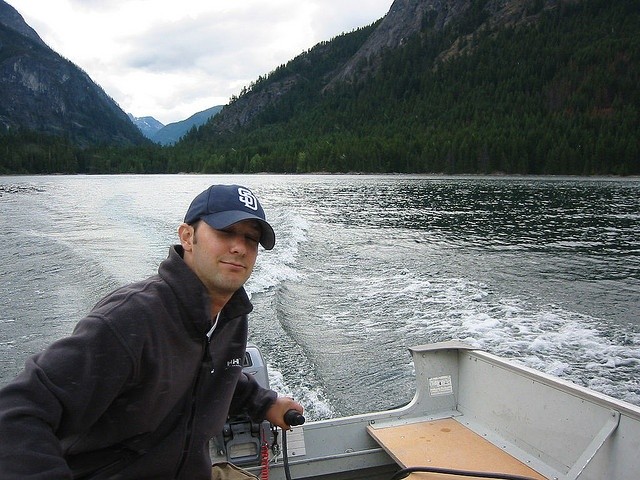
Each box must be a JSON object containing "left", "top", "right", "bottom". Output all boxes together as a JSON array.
[{"left": 0, "top": 185, "right": 303, "bottom": 480}]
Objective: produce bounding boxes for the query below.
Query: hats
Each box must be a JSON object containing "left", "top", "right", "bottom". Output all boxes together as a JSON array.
[{"left": 184, "top": 185, "right": 275, "bottom": 250}]
